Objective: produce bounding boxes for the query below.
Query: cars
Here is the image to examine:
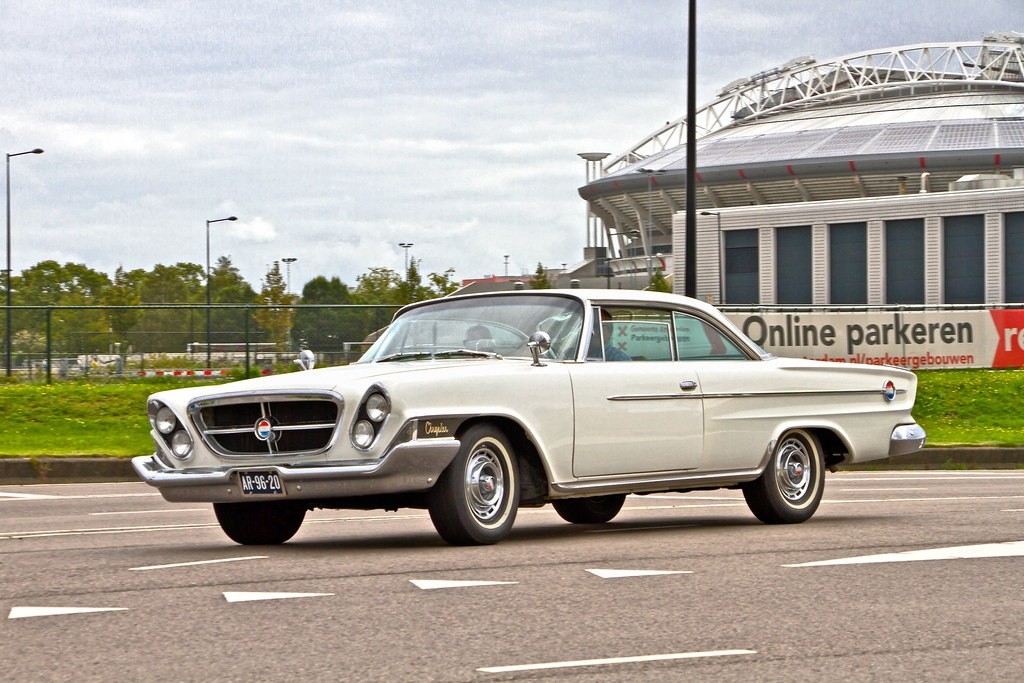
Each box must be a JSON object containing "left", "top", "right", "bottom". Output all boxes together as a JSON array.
[{"left": 129, "top": 289, "right": 927, "bottom": 547}]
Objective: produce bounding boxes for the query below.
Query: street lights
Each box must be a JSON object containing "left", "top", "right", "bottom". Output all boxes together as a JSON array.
[
  {"left": 398, "top": 242, "right": 413, "bottom": 281},
  {"left": 206, "top": 216, "right": 238, "bottom": 369},
  {"left": 282, "top": 258, "right": 297, "bottom": 293},
  {"left": 504, "top": 255, "right": 509, "bottom": 276},
  {"left": 562, "top": 263, "right": 567, "bottom": 272},
  {"left": 6, "top": 148, "right": 44, "bottom": 376}
]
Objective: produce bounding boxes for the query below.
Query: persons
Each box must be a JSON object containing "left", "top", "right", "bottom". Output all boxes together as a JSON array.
[
  {"left": 563, "top": 309, "right": 632, "bottom": 362},
  {"left": 462, "top": 327, "right": 493, "bottom": 357},
  {"left": 90, "top": 353, "right": 124, "bottom": 374}
]
